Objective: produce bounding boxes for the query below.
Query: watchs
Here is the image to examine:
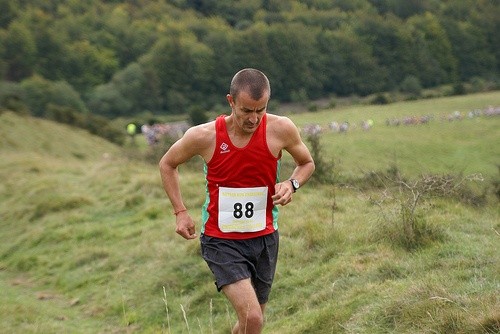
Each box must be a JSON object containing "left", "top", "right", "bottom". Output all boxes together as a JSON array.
[{"left": 288, "top": 177, "right": 300, "bottom": 193}]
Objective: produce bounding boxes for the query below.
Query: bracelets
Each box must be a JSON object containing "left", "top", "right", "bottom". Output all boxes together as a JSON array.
[{"left": 173, "top": 209, "right": 187, "bottom": 215}]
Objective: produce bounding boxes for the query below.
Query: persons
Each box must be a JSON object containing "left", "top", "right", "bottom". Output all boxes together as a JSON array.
[{"left": 158, "top": 68, "right": 316, "bottom": 334}]
